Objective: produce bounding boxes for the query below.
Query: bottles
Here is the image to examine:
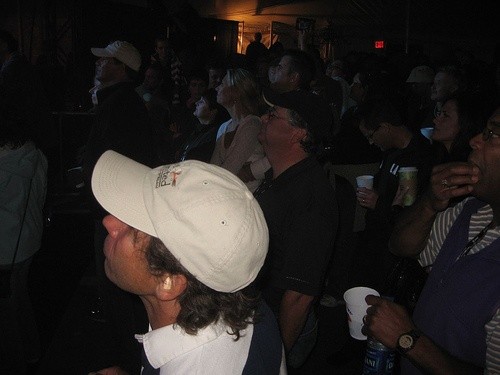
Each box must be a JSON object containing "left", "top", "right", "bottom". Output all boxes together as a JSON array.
[{"left": 362, "top": 296, "right": 401, "bottom": 374}]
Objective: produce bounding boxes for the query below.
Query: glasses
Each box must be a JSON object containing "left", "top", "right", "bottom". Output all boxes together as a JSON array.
[
  {"left": 267, "top": 110, "right": 293, "bottom": 122},
  {"left": 366, "top": 125, "right": 381, "bottom": 142},
  {"left": 99, "top": 58, "right": 119, "bottom": 66},
  {"left": 482, "top": 128, "right": 500, "bottom": 141}
]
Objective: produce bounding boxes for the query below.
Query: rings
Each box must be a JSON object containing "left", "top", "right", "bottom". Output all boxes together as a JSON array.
[
  {"left": 441, "top": 178, "right": 451, "bottom": 187},
  {"left": 363, "top": 198, "right": 365, "bottom": 203}
]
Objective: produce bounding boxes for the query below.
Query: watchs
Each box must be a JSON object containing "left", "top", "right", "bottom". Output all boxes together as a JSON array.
[{"left": 395, "top": 328, "right": 423, "bottom": 354}]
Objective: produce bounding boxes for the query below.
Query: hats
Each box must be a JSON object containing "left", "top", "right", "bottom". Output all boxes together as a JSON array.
[
  {"left": 91, "top": 40, "right": 141, "bottom": 71},
  {"left": 262, "top": 88, "right": 336, "bottom": 140},
  {"left": 405, "top": 66, "right": 435, "bottom": 83},
  {"left": 91, "top": 149, "right": 269, "bottom": 293}
]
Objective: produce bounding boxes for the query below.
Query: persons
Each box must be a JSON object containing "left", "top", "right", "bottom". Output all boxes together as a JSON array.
[
  {"left": 0, "top": 33, "right": 500, "bottom": 345},
  {"left": 251, "top": 87, "right": 347, "bottom": 375},
  {"left": 0, "top": 92, "right": 42, "bottom": 370},
  {"left": 90, "top": 150, "right": 287, "bottom": 375},
  {"left": 363, "top": 108, "right": 500, "bottom": 375}
]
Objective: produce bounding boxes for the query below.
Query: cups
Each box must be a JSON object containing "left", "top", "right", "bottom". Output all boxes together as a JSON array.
[
  {"left": 398, "top": 166, "right": 418, "bottom": 208},
  {"left": 420, "top": 127, "right": 434, "bottom": 141},
  {"left": 343, "top": 286, "right": 379, "bottom": 340},
  {"left": 356, "top": 176, "right": 374, "bottom": 201}
]
